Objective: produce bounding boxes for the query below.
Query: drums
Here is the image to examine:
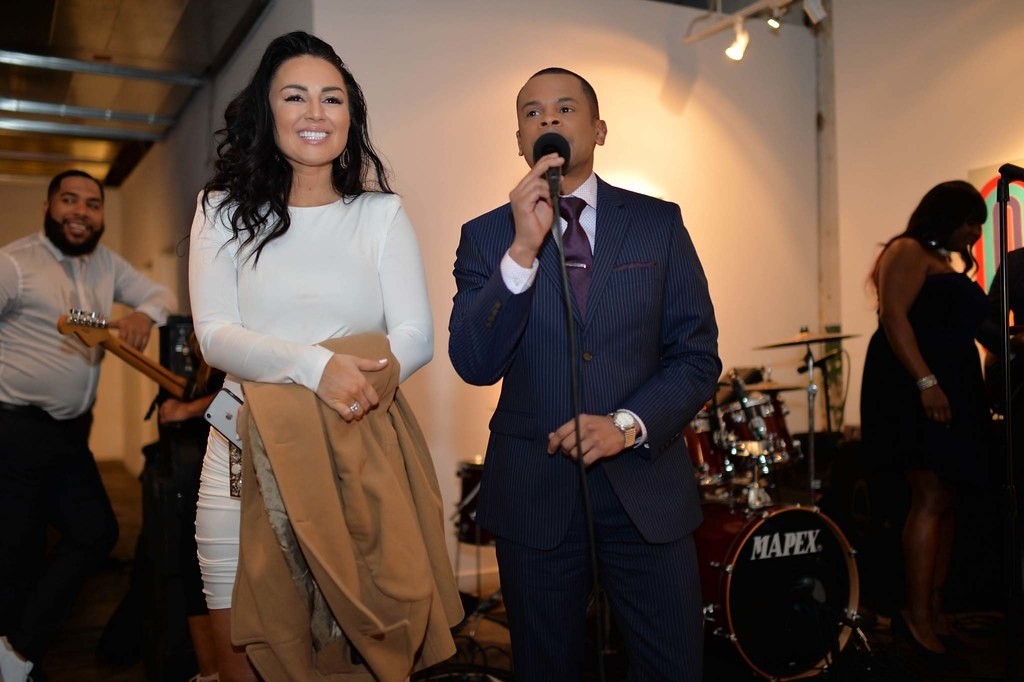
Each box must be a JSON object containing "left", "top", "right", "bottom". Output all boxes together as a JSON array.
[
  {"left": 682, "top": 394, "right": 801, "bottom": 491},
  {"left": 455, "top": 460, "right": 496, "bottom": 547},
  {"left": 693, "top": 497, "right": 860, "bottom": 682}
]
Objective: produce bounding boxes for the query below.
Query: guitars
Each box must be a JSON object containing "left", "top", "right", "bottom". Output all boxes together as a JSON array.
[{"left": 56, "top": 307, "right": 189, "bottom": 399}]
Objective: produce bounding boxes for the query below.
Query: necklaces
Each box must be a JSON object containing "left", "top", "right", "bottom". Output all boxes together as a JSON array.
[{"left": 929, "top": 241, "right": 947, "bottom": 256}]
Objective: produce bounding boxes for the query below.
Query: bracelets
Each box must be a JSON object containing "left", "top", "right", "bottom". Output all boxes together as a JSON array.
[{"left": 917, "top": 374, "right": 938, "bottom": 391}]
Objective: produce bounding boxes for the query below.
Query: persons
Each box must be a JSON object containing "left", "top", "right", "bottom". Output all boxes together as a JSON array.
[
  {"left": 159, "top": 331, "right": 227, "bottom": 682},
  {"left": 0, "top": 168, "right": 174, "bottom": 682},
  {"left": 448, "top": 67, "right": 723, "bottom": 682},
  {"left": 189, "top": 30, "right": 435, "bottom": 682},
  {"left": 859, "top": 180, "right": 1002, "bottom": 665}
]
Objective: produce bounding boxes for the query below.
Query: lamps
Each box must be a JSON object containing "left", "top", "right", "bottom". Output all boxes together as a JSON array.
[
  {"left": 802, "top": 0, "right": 827, "bottom": 25},
  {"left": 725, "top": 16, "right": 750, "bottom": 61},
  {"left": 767, "top": 8, "right": 784, "bottom": 29}
]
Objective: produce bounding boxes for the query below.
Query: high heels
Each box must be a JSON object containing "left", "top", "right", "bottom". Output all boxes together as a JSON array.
[
  {"left": 936, "top": 633, "right": 972, "bottom": 655},
  {"left": 891, "top": 607, "right": 970, "bottom": 674}
]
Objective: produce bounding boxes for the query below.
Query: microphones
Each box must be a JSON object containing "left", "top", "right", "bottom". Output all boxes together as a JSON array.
[
  {"left": 532, "top": 132, "right": 571, "bottom": 200},
  {"left": 794, "top": 575, "right": 814, "bottom": 595},
  {"left": 797, "top": 348, "right": 842, "bottom": 374}
]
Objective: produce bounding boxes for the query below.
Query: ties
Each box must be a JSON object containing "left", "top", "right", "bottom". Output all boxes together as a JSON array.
[{"left": 554, "top": 196, "right": 595, "bottom": 324}]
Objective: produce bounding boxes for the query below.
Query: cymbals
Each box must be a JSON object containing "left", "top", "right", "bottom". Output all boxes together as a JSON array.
[
  {"left": 753, "top": 332, "right": 863, "bottom": 351},
  {"left": 743, "top": 381, "right": 809, "bottom": 392}
]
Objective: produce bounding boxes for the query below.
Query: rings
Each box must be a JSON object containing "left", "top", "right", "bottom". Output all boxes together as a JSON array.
[{"left": 350, "top": 401, "right": 359, "bottom": 412}]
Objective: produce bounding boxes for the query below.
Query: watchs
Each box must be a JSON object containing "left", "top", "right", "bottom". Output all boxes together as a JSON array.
[{"left": 613, "top": 411, "right": 636, "bottom": 447}]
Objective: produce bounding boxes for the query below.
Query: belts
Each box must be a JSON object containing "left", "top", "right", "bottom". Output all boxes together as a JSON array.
[{"left": 0, "top": 402, "right": 95, "bottom": 426}]
